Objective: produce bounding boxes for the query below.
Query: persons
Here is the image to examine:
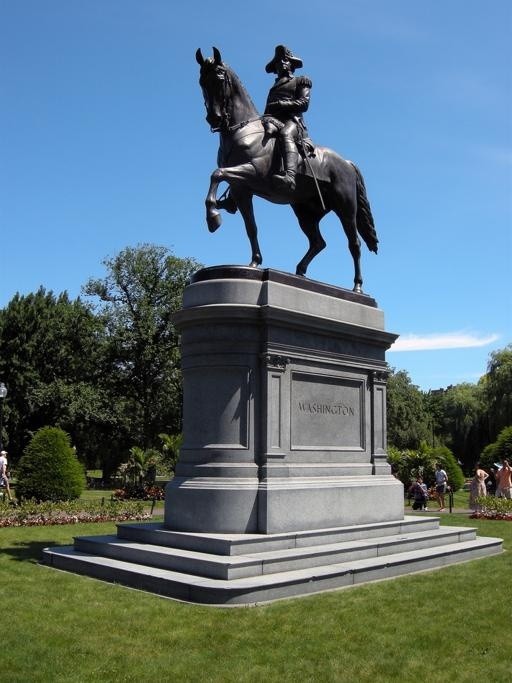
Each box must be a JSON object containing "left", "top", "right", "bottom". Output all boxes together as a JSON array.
[
  {"left": 0, "top": 451, "right": 15, "bottom": 502},
  {"left": 434, "top": 461, "right": 448, "bottom": 512},
  {"left": 264, "top": 44, "right": 312, "bottom": 191},
  {"left": 469, "top": 457, "right": 512, "bottom": 515},
  {"left": 407, "top": 476, "right": 429, "bottom": 512}
]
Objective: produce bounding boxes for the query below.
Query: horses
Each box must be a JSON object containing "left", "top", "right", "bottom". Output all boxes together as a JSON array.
[{"left": 195, "top": 46, "right": 378, "bottom": 293}]
